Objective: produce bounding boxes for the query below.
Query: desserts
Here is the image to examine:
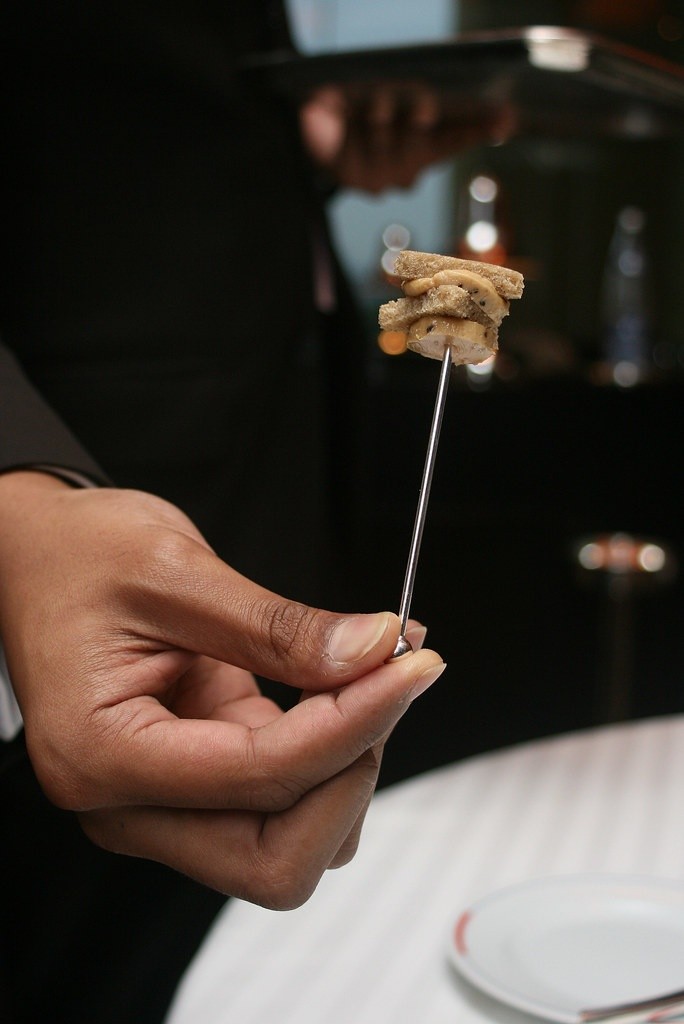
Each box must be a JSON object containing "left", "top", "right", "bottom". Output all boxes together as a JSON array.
[{"left": 376, "top": 251, "right": 525, "bottom": 366}]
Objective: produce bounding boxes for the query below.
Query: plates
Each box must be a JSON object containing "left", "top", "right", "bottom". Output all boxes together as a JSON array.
[{"left": 445, "top": 881, "right": 684, "bottom": 1024}]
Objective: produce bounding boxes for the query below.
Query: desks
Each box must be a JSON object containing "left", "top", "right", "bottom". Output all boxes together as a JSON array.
[{"left": 173, "top": 713, "right": 684, "bottom": 1024}]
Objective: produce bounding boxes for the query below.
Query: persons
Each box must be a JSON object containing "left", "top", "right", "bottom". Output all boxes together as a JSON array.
[{"left": 0, "top": 344, "right": 447, "bottom": 911}]
